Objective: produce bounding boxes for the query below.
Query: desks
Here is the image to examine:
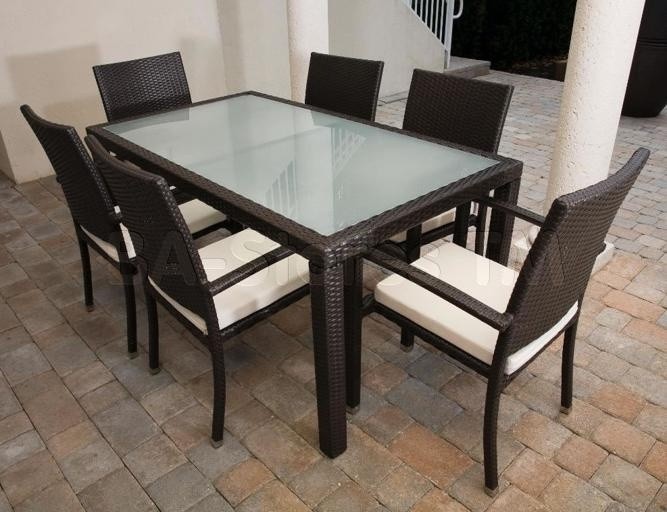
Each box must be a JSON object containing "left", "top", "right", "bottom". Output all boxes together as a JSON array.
[{"left": 87, "top": 91, "right": 525, "bottom": 460}]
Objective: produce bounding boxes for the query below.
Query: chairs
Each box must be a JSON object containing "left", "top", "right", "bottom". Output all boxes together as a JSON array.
[
  {"left": 84, "top": 135, "right": 310, "bottom": 451},
  {"left": 303, "top": 52, "right": 386, "bottom": 120},
  {"left": 91, "top": 51, "right": 193, "bottom": 121},
  {"left": 22, "top": 103, "right": 245, "bottom": 360},
  {"left": 360, "top": 71, "right": 514, "bottom": 352},
  {"left": 344, "top": 148, "right": 651, "bottom": 494}
]
